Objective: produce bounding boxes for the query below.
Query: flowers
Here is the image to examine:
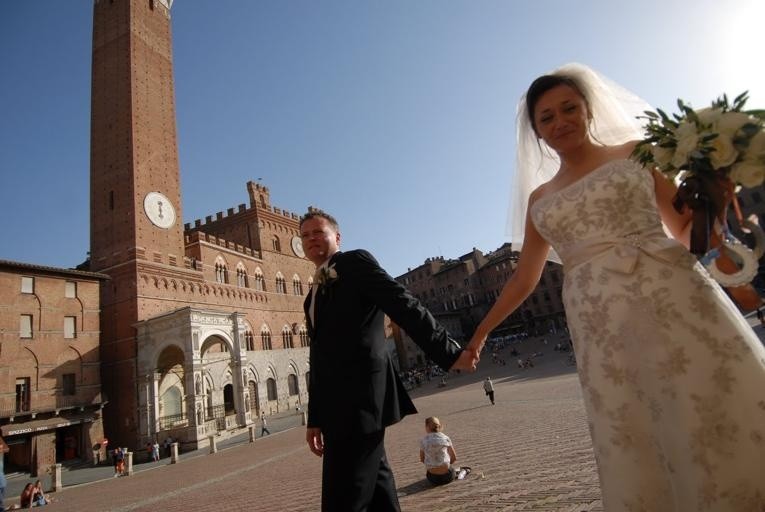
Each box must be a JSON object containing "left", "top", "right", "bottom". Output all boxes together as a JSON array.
[{"left": 628, "top": 90, "right": 765, "bottom": 254}]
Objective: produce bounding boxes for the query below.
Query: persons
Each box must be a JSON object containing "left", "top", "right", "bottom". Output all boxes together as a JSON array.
[
  {"left": 483, "top": 377, "right": 495, "bottom": 404},
  {"left": 457, "top": 62, "right": 764, "bottom": 512},
  {"left": 295, "top": 207, "right": 477, "bottom": 512},
  {"left": 112, "top": 446, "right": 125, "bottom": 477},
  {"left": 28, "top": 480, "right": 51, "bottom": 509},
  {"left": 296, "top": 401, "right": 299, "bottom": 416},
  {"left": 261, "top": 417, "right": 270, "bottom": 436},
  {"left": 146, "top": 437, "right": 180, "bottom": 461},
  {"left": 396, "top": 315, "right": 575, "bottom": 386},
  {"left": 262, "top": 412, "right": 264, "bottom": 421},
  {"left": 419, "top": 415, "right": 457, "bottom": 484},
  {"left": 21, "top": 483, "right": 36, "bottom": 508}
]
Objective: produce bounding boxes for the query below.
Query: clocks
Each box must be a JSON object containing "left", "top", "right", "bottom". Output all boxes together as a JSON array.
[{"left": 144, "top": 192, "right": 176, "bottom": 229}]
